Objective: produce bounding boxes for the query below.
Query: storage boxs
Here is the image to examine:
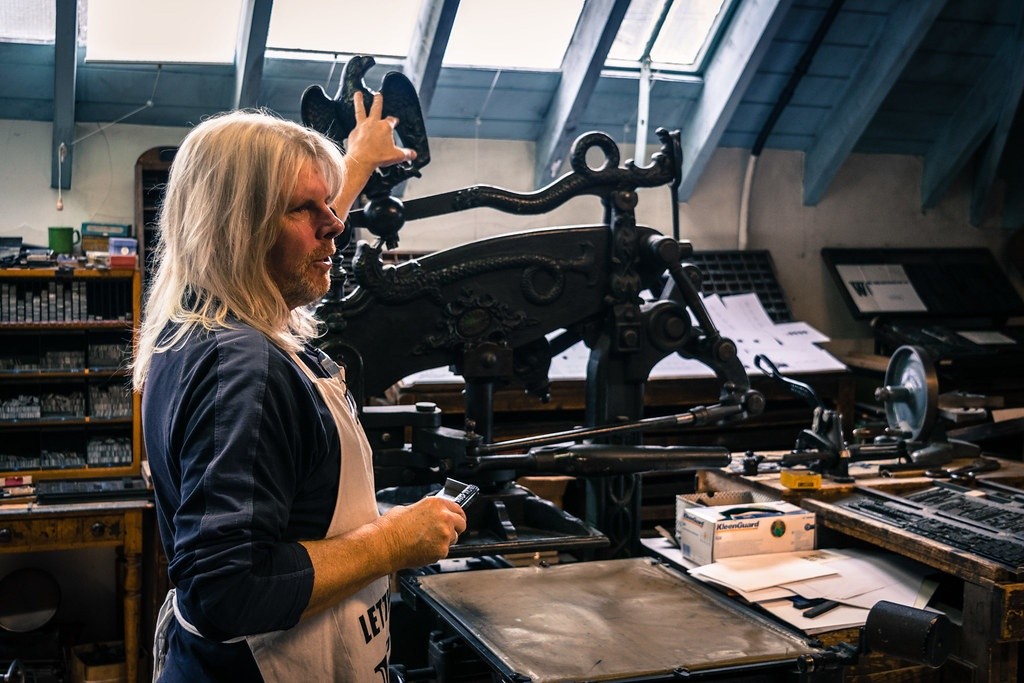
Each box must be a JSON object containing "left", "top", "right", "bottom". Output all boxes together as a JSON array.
[
  {"left": 676, "top": 490, "right": 816, "bottom": 566},
  {"left": 71, "top": 640, "right": 148, "bottom": 683},
  {"left": 81, "top": 222, "right": 138, "bottom": 269}
]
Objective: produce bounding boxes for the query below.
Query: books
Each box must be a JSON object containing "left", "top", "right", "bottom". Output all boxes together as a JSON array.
[{"left": 0, "top": 476, "right": 38, "bottom": 510}]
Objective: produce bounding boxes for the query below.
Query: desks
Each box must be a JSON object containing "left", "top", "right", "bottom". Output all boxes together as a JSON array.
[
  {"left": 838, "top": 352, "right": 892, "bottom": 427},
  {"left": 0, "top": 499, "right": 154, "bottom": 683},
  {"left": 700, "top": 449, "right": 1024, "bottom": 683}
]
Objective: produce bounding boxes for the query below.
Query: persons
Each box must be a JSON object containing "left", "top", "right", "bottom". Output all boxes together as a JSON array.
[{"left": 127, "top": 90, "right": 467, "bottom": 683}]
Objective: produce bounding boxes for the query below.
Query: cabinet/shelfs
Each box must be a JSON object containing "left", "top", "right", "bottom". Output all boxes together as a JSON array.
[
  {"left": 0, "top": 269, "right": 141, "bottom": 480},
  {"left": 387, "top": 366, "right": 855, "bottom": 568}
]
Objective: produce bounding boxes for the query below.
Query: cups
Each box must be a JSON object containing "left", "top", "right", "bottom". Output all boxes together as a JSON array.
[{"left": 48, "top": 227, "right": 81, "bottom": 257}]
